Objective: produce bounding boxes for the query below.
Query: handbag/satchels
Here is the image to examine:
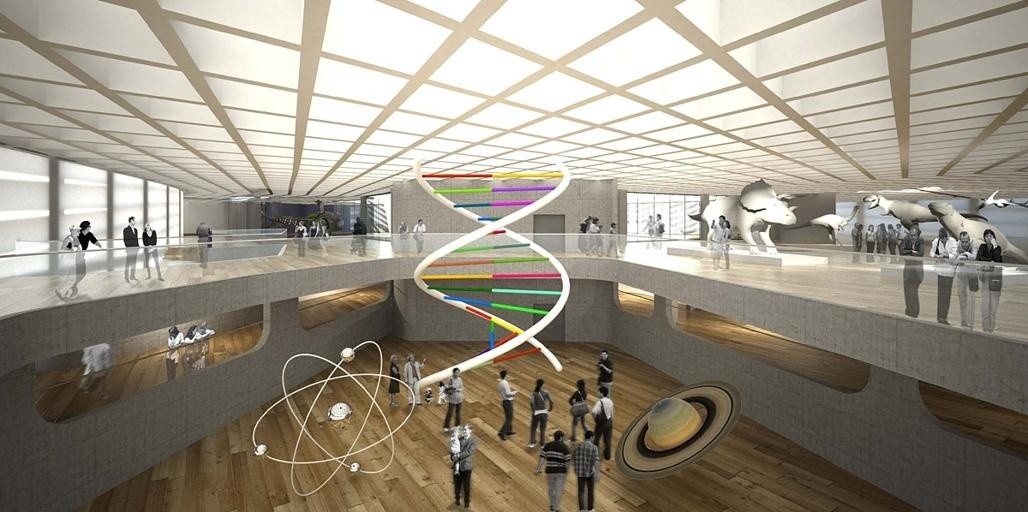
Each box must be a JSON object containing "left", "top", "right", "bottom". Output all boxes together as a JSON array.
[
  {"left": 595, "top": 414, "right": 608, "bottom": 423},
  {"left": 570, "top": 402, "right": 589, "bottom": 416}
]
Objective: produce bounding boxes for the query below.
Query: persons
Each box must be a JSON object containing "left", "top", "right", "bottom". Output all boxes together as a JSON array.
[
  {"left": 397, "top": 219, "right": 409, "bottom": 255},
  {"left": 78, "top": 343, "right": 113, "bottom": 399},
  {"left": 165, "top": 322, "right": 216, "bottom": 382},
  {"left": 497, "top": 370, "right": 519, "bottom": 441},
  {"left": 294, "top": 216, "right": 332, "bottom": 257},
  {"left": 413, "top": 220, "right": 427, "bottom": 252},
  {"left": 643, "top": 215, "right": 656, "bottom": 249},
  {"left": 388, "top": 350, "right": 477, "bottom": 508},
  {"left": 350, "top": 217, "right": 367, "bottom": 257},
  {"left": 527, "top": 350, "right": 614, "bottom": 511},
  {"left": 853, "top": 219, "right": 1006, "bottom": 333},
  {"left": 54, "top": 217, "right": 166, "bottom": 305},
  {"left": 577, "top": 215, "right": 621, "bottom": 259},
  {"left": 656, "top": 214, "right": 664, "bottom": 248},
  {"left": 711, "top": 215, "right": 726, "bottom": 270},
  {"left": 196, "top": 221, "right": 210, "bottom": 267},
  {"left": 721, "top": 220, "right": 731, "bottom": 272}
]
